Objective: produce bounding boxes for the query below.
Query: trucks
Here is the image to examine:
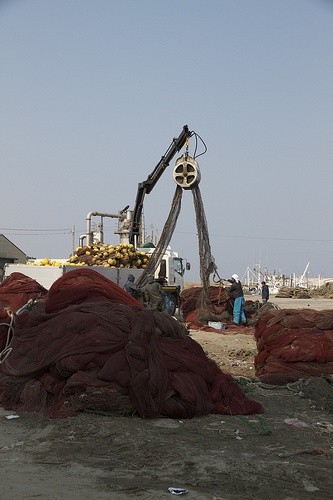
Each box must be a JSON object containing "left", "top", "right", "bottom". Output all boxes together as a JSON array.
[{"left": 3, "top": 245, "right": 191, "bottom": 318}]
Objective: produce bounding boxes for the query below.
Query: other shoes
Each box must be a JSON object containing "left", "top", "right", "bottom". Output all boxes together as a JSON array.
[
  {"left": 232, "top": 322, "right": 237, "bottom": 325},
  {"left": 240, "top": 321, "right": 247, "bottom": 325}
]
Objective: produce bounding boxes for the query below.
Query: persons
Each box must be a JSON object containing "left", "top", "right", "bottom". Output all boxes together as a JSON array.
[
  {"left": 124, "top": 273, "right": 168, "bottom": 312},
  {"left": 225, "top": 274, "right": 247, "bottom": 325},
  {"left": 261, "top": 281, "right": 269, "bottom": 302}
]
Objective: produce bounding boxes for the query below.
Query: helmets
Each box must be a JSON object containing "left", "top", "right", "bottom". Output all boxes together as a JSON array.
[{"left": 261, "top": 281, "right": 265, "bottom": 284}]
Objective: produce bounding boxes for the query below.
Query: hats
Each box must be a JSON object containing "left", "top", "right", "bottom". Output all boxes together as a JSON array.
[
  {"left": 128, "top": 274, "right": 135, "bottom": 282},
  {"left": 146, "top": 274, "right": 153, "bottom": 284},
  {"left": 232, "top": 274, "right": 240, "bottom": 284}
]
